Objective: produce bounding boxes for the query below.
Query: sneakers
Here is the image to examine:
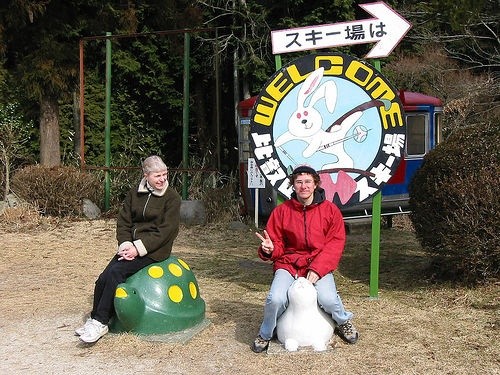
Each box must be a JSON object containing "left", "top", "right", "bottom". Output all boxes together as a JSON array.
[
  {"left": 79, "top": 319, "right": 108, "bottom": 342},
  {"left": 338, "top": 320, "right": 358, "bottom": 344},
  {"left": 75, "top": 317, "right": 93, "bottom": 336},
  {"left": 253, "top": 336, "right": 268, "bottom": 352}
]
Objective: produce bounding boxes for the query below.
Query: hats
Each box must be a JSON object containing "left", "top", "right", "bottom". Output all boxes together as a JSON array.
[
  {"left": 293, "top": 166, "right": 316, "bottom": 174},
  {"left": 143, "top": 156, "right": 167, "bottom": 174}
]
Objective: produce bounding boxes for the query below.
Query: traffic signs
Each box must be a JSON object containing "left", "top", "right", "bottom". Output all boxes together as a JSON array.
[{"left": 271, "top": 1, "right": 413, "bottom": 59}]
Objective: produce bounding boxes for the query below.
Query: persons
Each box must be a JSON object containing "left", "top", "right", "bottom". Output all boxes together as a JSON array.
[
  {"left": 74, "top": 155, "right": 181, "bottom": 343},
  {"left": 252, "top": 166, "right": 358, "bottom": 354}
]
